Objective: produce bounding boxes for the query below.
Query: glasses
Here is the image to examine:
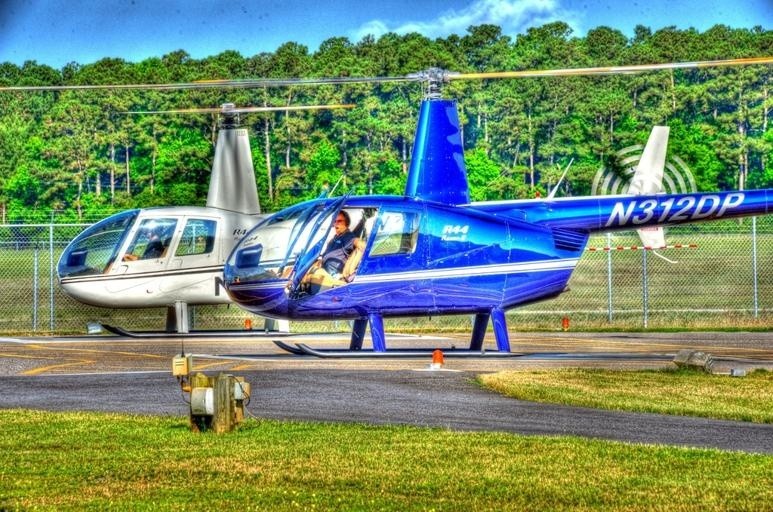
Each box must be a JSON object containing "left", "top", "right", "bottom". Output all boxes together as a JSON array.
[{"left": 334, "top": 219, "right": 346, "bottom": 223}]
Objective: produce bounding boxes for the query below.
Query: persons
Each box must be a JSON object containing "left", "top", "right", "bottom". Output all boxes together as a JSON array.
[
  {"left": 122, "top": 219, "right": 166, "bottom": 263},
  {"left": 283, "top": 211, "right": 368, "bottom": 290}
]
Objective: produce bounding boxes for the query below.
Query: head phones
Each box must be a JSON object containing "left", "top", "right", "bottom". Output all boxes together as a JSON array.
[{"left": 340, "top": 210, "right": 350, "bottom": 225}]
